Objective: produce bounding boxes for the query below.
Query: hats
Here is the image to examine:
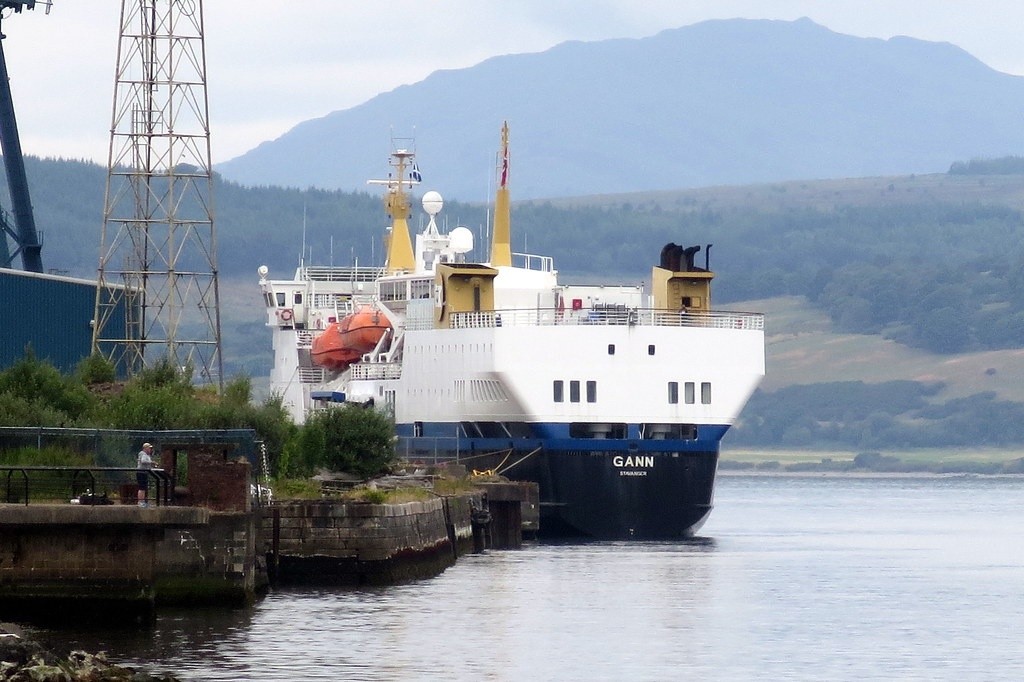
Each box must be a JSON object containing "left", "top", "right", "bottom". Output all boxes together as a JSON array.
[{"left": 143, "top": 443, "right": 153, "bottom": 447}]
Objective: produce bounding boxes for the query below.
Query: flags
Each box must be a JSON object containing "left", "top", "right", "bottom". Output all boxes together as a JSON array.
[{"left": 412, "top": 163, "right": 422, "bottom": 182}]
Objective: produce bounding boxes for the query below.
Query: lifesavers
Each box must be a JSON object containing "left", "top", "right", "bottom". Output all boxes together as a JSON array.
[{"left": 281, "top": 308, "right": 292, "bottom": 320}]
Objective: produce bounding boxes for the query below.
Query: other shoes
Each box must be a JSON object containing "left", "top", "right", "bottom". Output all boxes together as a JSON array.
[{"left": 138, "top": 499, "right": 147, "bottom": 508}]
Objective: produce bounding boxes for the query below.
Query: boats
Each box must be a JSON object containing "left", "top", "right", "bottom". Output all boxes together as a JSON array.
[{"left": 257, "top": 121, "right": 765, "bottom": 539}]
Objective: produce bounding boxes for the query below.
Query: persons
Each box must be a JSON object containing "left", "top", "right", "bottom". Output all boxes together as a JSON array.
[{"left": 136, "top": 443, "right": 158, "bottom": 507}]
